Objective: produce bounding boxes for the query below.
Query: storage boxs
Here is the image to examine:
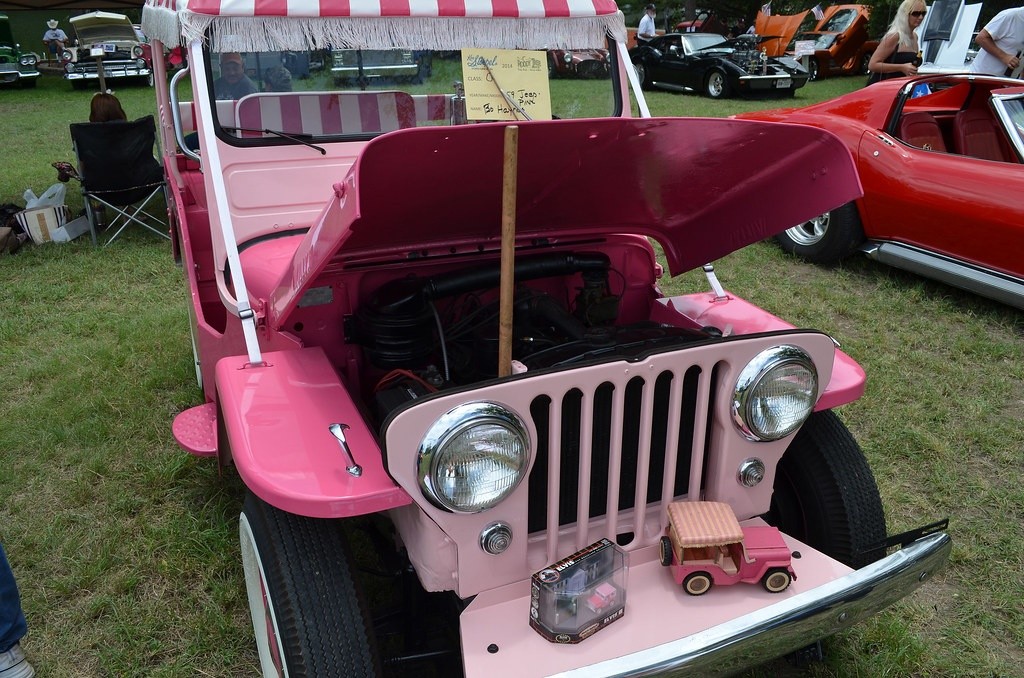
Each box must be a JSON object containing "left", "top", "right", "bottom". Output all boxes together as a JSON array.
[{"left": 51, "top": 215, "right": 89, "bottom": 243}]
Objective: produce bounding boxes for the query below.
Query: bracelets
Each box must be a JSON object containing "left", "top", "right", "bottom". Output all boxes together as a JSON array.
[{"left": 650, "top": 34, "right": 653, "bottom": 37}]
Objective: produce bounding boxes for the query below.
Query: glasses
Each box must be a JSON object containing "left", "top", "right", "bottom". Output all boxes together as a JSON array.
[
  {"left": 911, "top": 11, "right": 926, "bottom": 17},
  {"left": 652, "top": 8, "right": 658, "bottom": 12}
]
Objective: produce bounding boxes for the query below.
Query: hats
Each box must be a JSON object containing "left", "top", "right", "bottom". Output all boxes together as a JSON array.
[
  {"left": 222, "top": 52, "right": 242, "bottom": 65},
  {"left": 646, "top": 4, "right": 654, "bottom": 10},
  {"left": 265, "top": 65, "right": 292, "bottom": 92},
  {"left": 47, "top": 19, "right": 58, "bottom": 29}
]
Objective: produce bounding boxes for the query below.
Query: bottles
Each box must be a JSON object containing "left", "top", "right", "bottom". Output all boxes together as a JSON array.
[
  {"left": 910, "top": 50, "right": 923, "bottom": 68},
  {"left": 92, "top": 201, "right": 107, "bottom": 229},
  {"left": 1004, "top": 51, "right": 1022, "bottom": 77}
]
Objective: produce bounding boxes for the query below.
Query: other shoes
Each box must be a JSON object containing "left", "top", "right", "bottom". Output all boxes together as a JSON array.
[{"left": 124, "top": 215, "right": 148, "bottom": 225}]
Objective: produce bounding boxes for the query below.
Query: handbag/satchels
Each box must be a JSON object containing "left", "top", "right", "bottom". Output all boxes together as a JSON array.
[
  {"left": 13, "top": 204, "right": 69, "bottom": 246},
  {"left": 23, "top": 183, "right": 66, "bottom": 208}
]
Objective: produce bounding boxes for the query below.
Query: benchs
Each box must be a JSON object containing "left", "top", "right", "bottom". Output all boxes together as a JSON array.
[{"left": 236, "top": 91, "right": 417, "bottom": 136}]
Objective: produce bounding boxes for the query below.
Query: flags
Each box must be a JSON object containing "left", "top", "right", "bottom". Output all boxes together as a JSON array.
[
  {"left": 812, "top": 5, "right": 825, "bottom": 20},
  {"left": 762, "top": 3, "right": 770, "bottom": 16}
]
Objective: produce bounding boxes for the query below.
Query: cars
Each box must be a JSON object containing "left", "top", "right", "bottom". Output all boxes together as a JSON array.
[
  {"left": 719, "top": 71, "right": 1024, "bottom": 312},
  {"left": 327, "top": 44, "right": 432, "bottom": 85},
  {"left": 752, "top": 6, "right": 882, "bottom": 82},
  {"left": 515, "top": 43, "right": 612, "bottom": 80},
  {"left": 673, "top": 9, "right": 738, "bottom": 50},
  {"left": 136, "top": 0, "right": 959, "bottom": 678},
  {"left": 628, "top": 32, "right": 814, "bottom": 99},
  {"left": 65, "top": 12, "right": 172, "bottom": 89},
  {"left": 0, "top": 15, "right": 44, "bottom": 92}
]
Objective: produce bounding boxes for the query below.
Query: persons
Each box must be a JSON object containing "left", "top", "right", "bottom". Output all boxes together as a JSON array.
[
  {"left": 211, "top": 52, "right": 292, "bottom": 101},
  {"left": 43, "top": 19, "right": 68, "bottom": 63},
  {"left": 720, "top": 19, "right": 756, "bottom": 38},
  {"left": 637, "top": 4, "right": 658, "bottom": 91},
  {"left": 867, "top": 0, "right": 929, "bottom": 87},
  {"left": 966, "top": 7, "right": 1024, "bottom": 79},
  {"left": 77, "top": 93, "right": 147, "bottom": 224}
]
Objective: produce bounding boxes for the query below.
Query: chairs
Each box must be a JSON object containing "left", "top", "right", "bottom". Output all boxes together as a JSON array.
[
  {"left": 53, "top": 115, "right": 172, "bottom": 248},
  {"left": 895, "top": 111, "right": 948, "bottom": 152},
  {"left": 45, "top": 42, "right": 69, "bottom": 68},
  {"left": 953, "top": 109, "right": 1006, "bottom": 163}
]
